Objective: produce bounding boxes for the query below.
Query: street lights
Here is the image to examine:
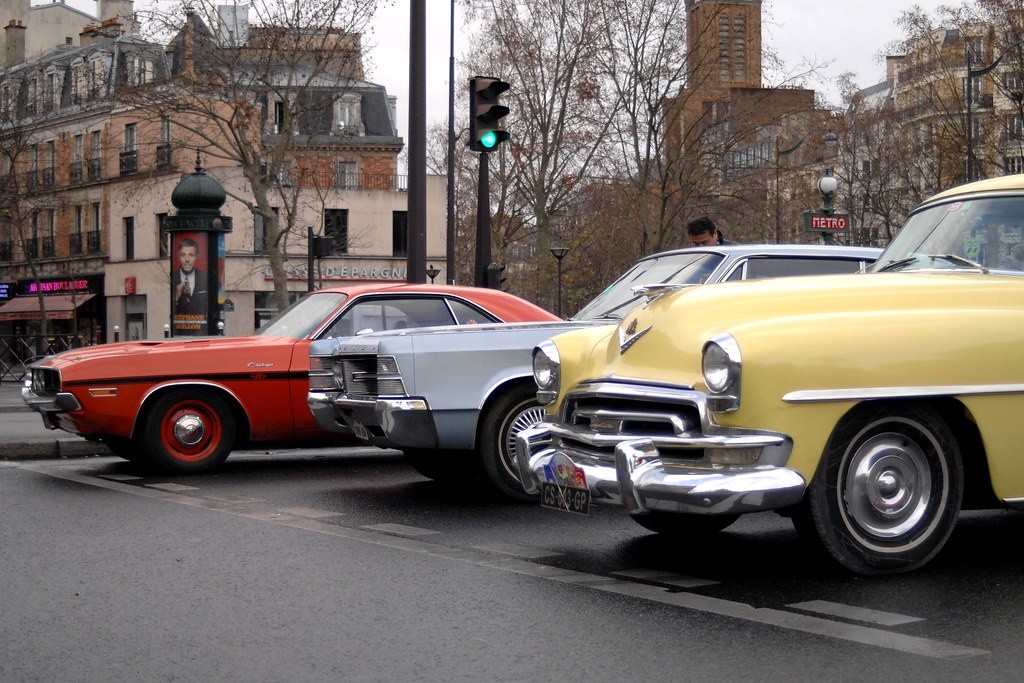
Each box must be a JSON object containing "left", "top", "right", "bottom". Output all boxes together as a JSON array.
[{"left": 552, "top": 247, "right": 570, "bottom": 318}]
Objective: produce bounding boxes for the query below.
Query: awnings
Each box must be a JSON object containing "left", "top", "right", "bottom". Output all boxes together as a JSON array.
[{"left": 0, "top": 294, "right": 97, "bottom": 320}]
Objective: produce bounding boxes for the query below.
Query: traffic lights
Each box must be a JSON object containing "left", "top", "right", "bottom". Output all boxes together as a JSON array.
[
  {"left": 312, "top": 234, "right": 334, "bottom": 259},
  {"left": 467, "top": 75, "right": 511, "bottom": 153}
]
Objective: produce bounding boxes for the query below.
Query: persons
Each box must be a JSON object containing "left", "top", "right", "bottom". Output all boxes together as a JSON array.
[
  {"left": 687, "top": 216, "right": 741, "bottom": 247},
  {"left": 170, "top": 239, "right": 210, "bottom": 315}
]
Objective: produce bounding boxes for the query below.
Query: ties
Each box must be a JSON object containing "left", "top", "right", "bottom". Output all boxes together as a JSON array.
[{"left": 185, "top": 277, "right": 192, "bottom": 301}]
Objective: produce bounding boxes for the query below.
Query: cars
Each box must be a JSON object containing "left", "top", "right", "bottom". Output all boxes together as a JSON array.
[
  {"left": 309, "top": 242, "right": 895, "bottom": 509},
  {"left": 21, "top": 282, "right": 562, "bottom": 478},
  {"left": 512, "top": 174, "right": 1024, "bottom": 577}
]
